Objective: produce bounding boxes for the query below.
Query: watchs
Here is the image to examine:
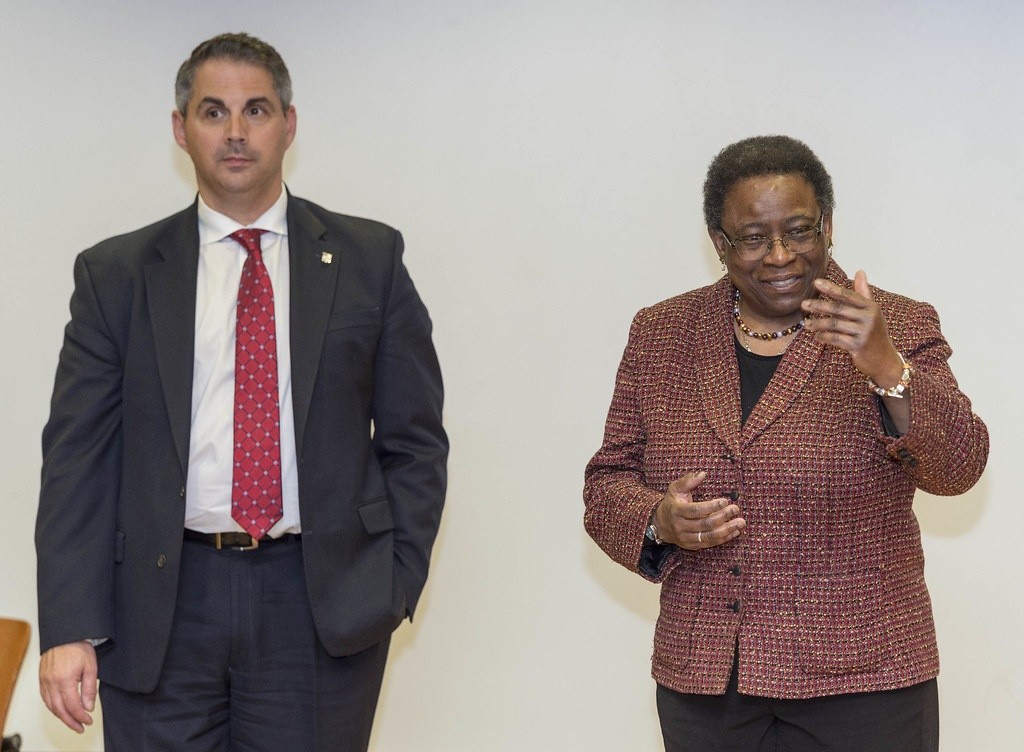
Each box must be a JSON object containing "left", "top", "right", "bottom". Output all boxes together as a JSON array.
[{"left": 645, "top": 519, "right": 663, "bottom": 544}]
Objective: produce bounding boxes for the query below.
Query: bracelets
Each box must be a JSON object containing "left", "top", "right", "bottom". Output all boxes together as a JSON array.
[{"left": 865, "top": 353, "right": 913, "bottom": 399}]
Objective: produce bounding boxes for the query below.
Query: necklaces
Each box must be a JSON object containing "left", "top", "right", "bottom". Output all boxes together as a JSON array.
[{"left": 732, "top": 289, "right": 808, "bottom": 354}]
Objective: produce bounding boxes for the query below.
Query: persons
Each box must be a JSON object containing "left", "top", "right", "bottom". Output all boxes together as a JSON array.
[
  {"left": 583, "top": 136, "right": 989, "bottom": 752},
  {"left": 32, "top": 36, "right": 448, "bottom": 752}
]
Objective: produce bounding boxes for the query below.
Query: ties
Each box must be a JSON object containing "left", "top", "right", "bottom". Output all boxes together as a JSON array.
[{"left": 229, "top": 228, "right": 284, "bottom": 538}]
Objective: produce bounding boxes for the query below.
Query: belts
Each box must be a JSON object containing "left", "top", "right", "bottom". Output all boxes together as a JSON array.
[{"left": 185, "top": 530, "right": 282, "bottom": 547}]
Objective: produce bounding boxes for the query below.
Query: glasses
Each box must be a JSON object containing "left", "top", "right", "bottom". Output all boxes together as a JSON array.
[{"left": 717, "top": 210, "right": 823, "bottom": 260}]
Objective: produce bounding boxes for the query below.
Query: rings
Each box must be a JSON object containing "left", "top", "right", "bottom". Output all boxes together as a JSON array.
[{"left": 698, "top": 532, "right": 702, "bottom": 542}]
